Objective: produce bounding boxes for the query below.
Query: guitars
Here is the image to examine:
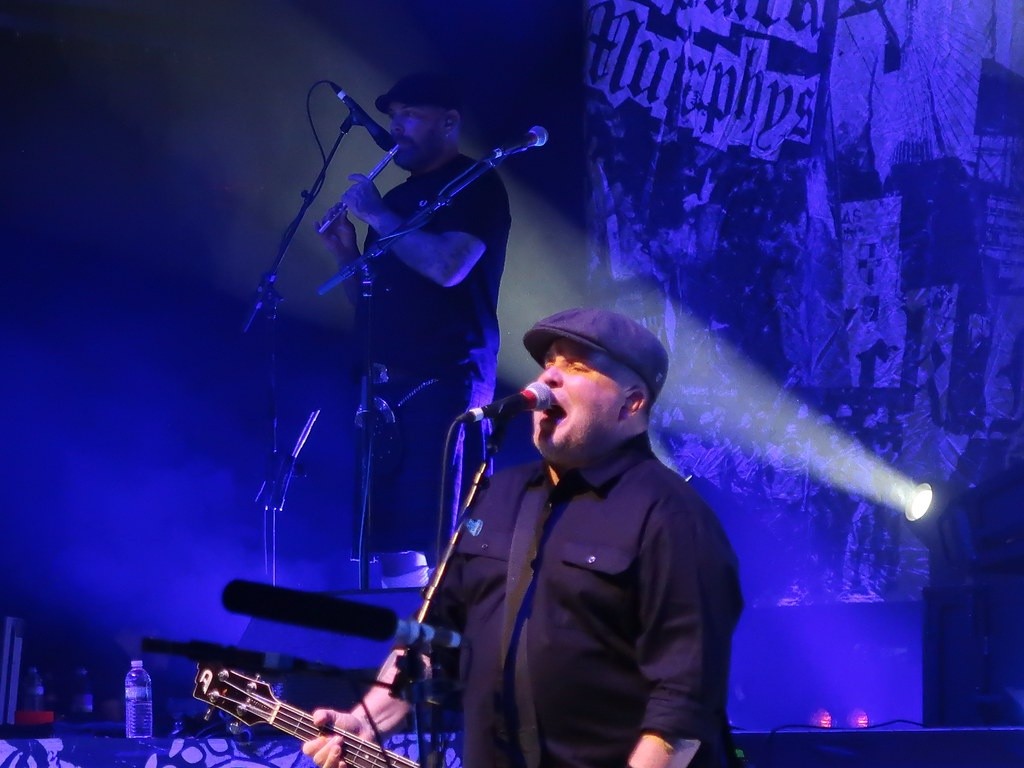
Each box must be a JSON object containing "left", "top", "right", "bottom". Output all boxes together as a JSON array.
[{"left": 192, "top": 658, "right": 419, "bottom": 767}]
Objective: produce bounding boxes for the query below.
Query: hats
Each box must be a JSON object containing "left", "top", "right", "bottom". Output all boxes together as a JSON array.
[
  {"left": 375, "top": 69, "right": 460, "bottom": 110},
  {"left": 522, "top": 307, "right": 669, "bottom": 401}
]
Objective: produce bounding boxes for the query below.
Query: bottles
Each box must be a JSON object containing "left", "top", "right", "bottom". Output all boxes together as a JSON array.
[
  {"left": 22, "top": 668, "right": 45, "bottom": 711},
  {"left": 125, "top": 661, "right": 153, "bottom": 738},
  {"left": 76, "top": 669, "right": 93, "bottom": 712}
]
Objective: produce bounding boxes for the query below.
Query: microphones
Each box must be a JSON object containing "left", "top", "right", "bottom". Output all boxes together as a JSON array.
[
  {"left": 330, "top": 82, "right": 397, "bottom": 152},
  {"left": 222, "top": 578, "right": 470, "bottom": 650},
  {"left": 457, "top": 380, "right": 554, "bottom": 423},
  {"left": 484, "top": 126, "right": 549, "bottom": 161}
]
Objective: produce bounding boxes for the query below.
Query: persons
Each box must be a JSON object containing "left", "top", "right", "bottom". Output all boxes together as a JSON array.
[
  {"left": 313, "top": 70, "right": 512, "bottom": 588},
  {"left": 302, "top": 308, "right": 745, "bottom": 768}
]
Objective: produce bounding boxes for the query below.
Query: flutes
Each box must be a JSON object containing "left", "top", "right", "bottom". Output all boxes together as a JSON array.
[{"left": 318, "top": 143, "right": 400, "bottom": 235}]
